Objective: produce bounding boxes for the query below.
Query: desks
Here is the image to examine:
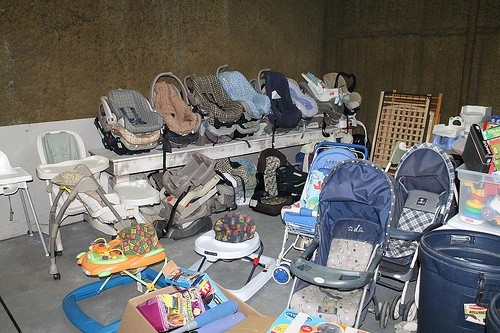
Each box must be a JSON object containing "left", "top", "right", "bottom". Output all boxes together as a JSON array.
[{"left": 88, "top": 126, "right": 353, "bottom": 193}]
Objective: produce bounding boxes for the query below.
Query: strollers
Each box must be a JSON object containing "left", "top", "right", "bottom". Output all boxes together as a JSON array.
[
  {"left": 374, "top": 142, "right": 459, "bottom": 322},
  {"left": 286, "top": 158, "right": 391, "bottom": 329},
  {"left": 271, "top": 141, "right": 369, "bottom": 285}
]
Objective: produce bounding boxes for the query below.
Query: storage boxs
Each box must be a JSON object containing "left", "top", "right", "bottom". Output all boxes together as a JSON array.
[
  {"left": 117, "top": 281, "right": 371, "bottom": 333},
  {"left": 454, "top": 162, "right": 500, "bottom": 234}
]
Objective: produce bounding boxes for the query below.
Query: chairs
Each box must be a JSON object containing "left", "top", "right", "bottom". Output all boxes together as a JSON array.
[
  {"left": 0, "top": 151, "right": 49, "bottom": 257},
  {"left": 370, "top": 90, "right": 443, "bottom": 177},
  {"left": 35, "top": 129, "right": 126, "bottom": 257}
]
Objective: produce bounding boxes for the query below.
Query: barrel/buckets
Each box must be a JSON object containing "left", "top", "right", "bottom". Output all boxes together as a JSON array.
[{"left": 418, "top": 230, "right": 500, "bottom": 333}]
